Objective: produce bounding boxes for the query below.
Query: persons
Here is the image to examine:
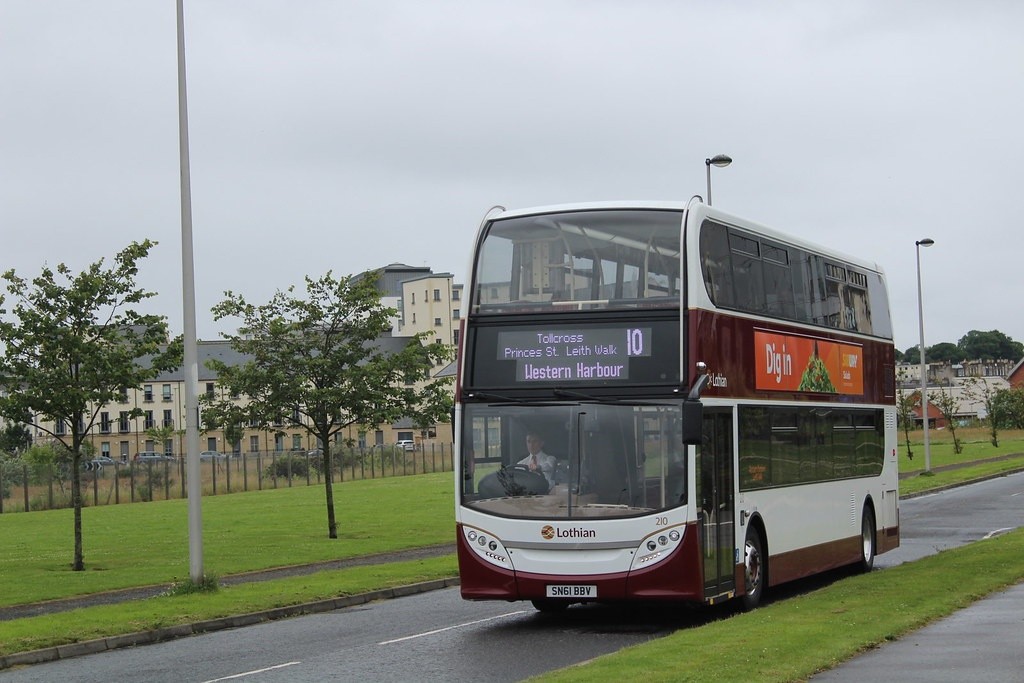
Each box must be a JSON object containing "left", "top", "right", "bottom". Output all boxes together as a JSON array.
[{"left": 514, "top": 431, "right": 556, "bottom": 489}]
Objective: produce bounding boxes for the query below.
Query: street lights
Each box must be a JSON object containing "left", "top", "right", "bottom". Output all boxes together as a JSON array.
[
  {"left": 915, "top": 238, "right": 936, "bottom": 473},
  {"left": 704, "top": 153, "right": 733, "bottom": 206}
]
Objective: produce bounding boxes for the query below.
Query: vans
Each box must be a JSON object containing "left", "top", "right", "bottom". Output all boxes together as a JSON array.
[{"left": 455, "top": 195, "right": 901, "bottom": 619}]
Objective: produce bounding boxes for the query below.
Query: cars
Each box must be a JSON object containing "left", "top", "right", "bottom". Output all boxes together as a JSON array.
[
  {"left": 200, "top": 451, "right": 233, "bottom": 462},
  {"left": 395, "top": 440, "right": 414, "bottom": 452},
  {"left": 134, "top": 451, "right": 174, "bottom": 463},
  {"left": 92, "top": 456, "right": 125, "bottom": 465}
]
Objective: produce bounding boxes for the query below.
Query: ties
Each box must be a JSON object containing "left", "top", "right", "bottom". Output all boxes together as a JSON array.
[{"left": 531, "top": 456, "right": 537, "bottom": 468}]
